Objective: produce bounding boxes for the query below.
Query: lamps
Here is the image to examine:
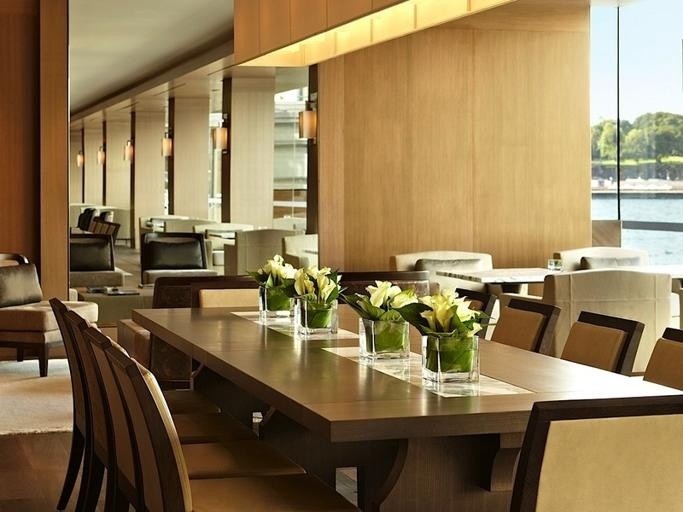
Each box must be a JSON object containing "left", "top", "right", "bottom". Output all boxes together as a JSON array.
[
  {"left": 95, "top": 150, "right": 105, "bottom": 164},
  {"left": 160, "top": 137, "right": 172, "bottom": 157},
  {"left": 298, "top": 110, "right": 316, "bottom": 145},
  {"left": 123, "top": 144, "right": 133, "bottom": 161},
  {"left": 210, "top": 127, "right": 227, "bottom": 151},
  {"left": 75, "top": 153, "right": 82, "bottom": 167}
]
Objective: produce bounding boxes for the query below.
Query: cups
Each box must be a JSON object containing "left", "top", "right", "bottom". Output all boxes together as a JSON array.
[{"left": 547, "top": 259, "right": 568, "bottom": 274}]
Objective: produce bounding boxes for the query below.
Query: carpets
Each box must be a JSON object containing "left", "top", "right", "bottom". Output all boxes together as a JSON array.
[{"left": 0, "top": 357, "right": 74, "bottom": 434}]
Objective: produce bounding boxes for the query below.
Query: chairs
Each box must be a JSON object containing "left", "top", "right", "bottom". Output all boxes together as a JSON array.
[
  {"left": 49, "top": 246, "right": 683, "bottom": 512},
  {"left": 0, "top": 252, "right": 100, "bottom": 379},
  {"left": 67, "top": 206, "right": 318, "bottom": 326}
]
